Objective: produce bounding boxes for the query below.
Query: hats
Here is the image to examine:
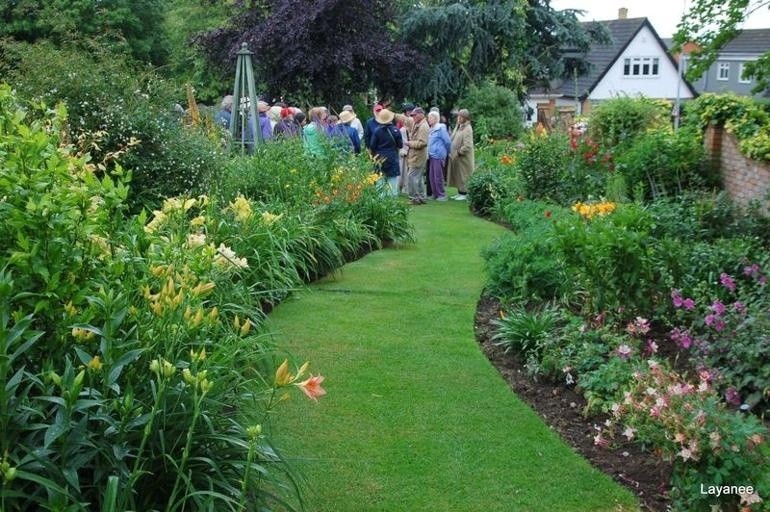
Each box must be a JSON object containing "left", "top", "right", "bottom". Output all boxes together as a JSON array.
[{"left": 221, "top": 94, "right": 471, "bottom": 127}]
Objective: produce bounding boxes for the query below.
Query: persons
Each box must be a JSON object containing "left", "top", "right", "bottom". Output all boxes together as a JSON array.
[{"left": 216, "top": 94, "right": 476, "bottom": 203}]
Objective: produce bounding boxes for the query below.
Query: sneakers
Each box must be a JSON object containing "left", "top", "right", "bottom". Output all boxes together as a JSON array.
[{"left": 409, "top": 193, "right": 471, "bottom": 206}]
